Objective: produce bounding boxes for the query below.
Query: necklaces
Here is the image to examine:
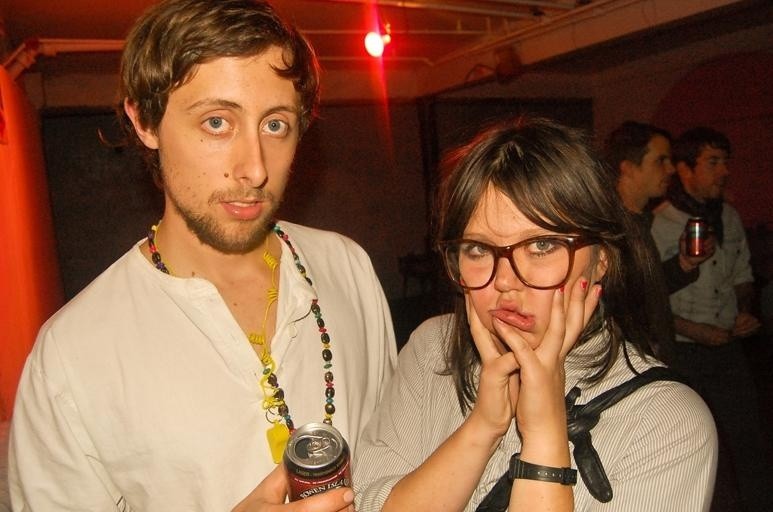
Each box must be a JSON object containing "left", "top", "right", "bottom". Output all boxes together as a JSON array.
[{"left": 147, "top": 215, "right": 337, "bottom": 436}]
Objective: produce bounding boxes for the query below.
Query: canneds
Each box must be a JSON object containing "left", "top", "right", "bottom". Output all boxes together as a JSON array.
[
  {"left": 686, "top": 217, "right": 707, "bottom": 256},
  {"left": 282, "top": 422, "right": 352, "bottom": 502}
]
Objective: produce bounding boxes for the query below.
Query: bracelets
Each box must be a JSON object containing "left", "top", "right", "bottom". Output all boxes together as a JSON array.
[{"left": 505, "top": 450, "right": 579, "bottom": 487}]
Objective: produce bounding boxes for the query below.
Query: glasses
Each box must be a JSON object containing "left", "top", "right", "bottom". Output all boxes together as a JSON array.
[{"left": 434, "top": 235, "right": 601, "bottom": 291}]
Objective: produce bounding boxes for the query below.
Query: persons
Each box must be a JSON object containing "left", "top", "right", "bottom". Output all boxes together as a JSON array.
[
  {"left": 349, "top": 117, "right": 721, "bottom": 511},
  {"left": 598, "top": 119, "right": 716, "bottom": 379},
  {"left": 5, "top": 0, "right": 398, "bottom": 510},
  {"left": 648, "top": 123, "right": 773, "bottom": 510}
]
